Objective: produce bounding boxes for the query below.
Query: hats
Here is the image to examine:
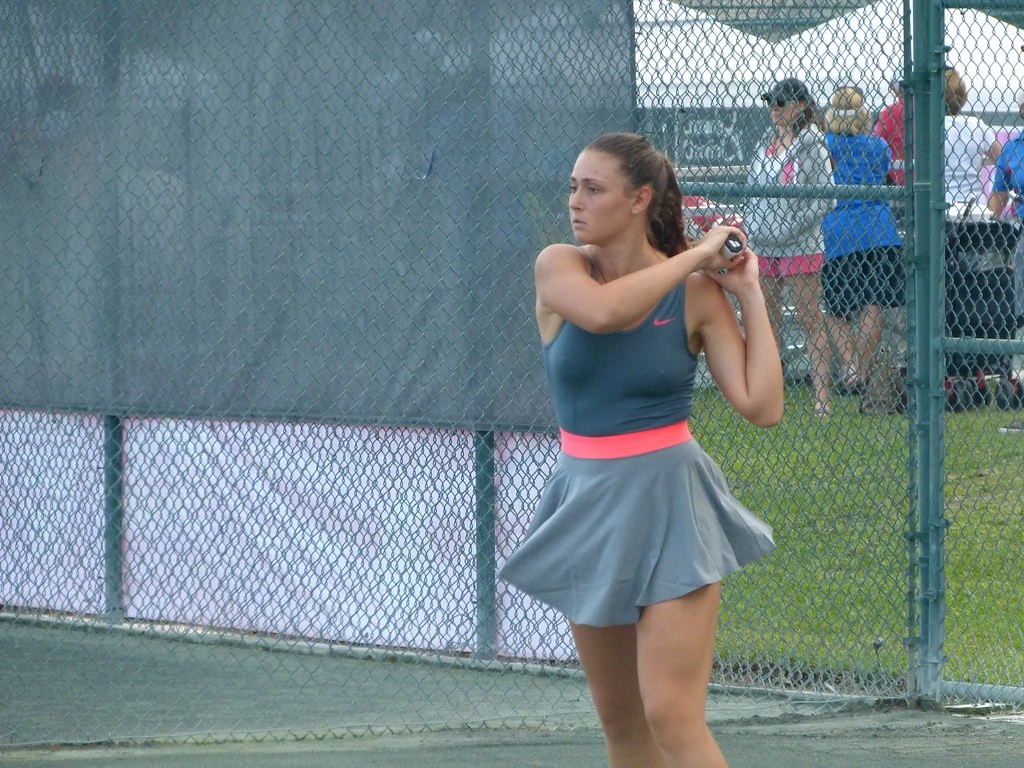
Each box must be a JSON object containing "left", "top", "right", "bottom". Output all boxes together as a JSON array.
[{"left": 761, "top": 78, "right": 809, "bottom": 100}]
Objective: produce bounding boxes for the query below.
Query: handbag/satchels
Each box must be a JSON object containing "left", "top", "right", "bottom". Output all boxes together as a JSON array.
[{"left": 859, "top": 347, "right": 907, "bottom": 414}]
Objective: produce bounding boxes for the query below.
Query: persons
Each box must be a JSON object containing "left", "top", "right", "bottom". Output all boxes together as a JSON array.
[
  {"left": 944, "top": 64, "right": 1006, "bottom": 407},
  {"left": 987, "top": 105, "right": 1022, "bottom": 388},
  {"left": 820, "top": 87, "right": 908, "bottom": 392},
  {"left": 869, "top": 71, "right": 914, "bottom": 187},
  {"left": 742, "top": 78, "right": 833, "bottom": 416},
  {"left": 490, "top": 130, "right": 789, "bottom": 768}
]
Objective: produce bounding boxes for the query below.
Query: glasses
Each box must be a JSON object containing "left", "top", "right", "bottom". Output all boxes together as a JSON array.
[{"left": 767, "top": 100, "right": 785, "bottom": 107}]
